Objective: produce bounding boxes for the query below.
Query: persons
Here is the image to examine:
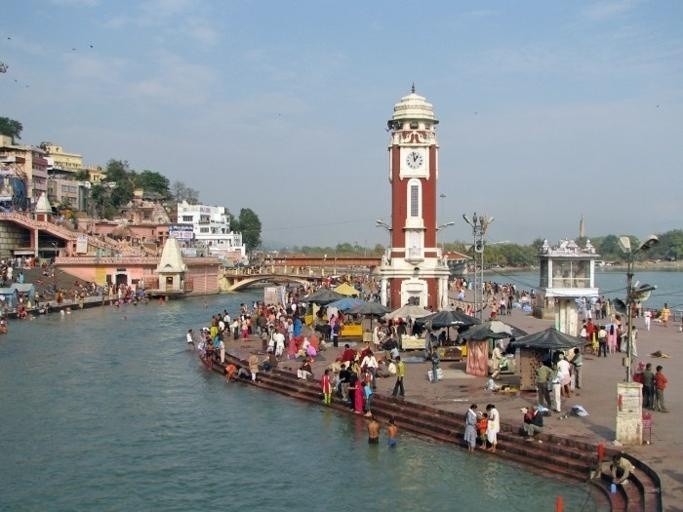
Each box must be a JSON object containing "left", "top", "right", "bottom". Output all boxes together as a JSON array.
[
  {"left": 384, "top": 419, "right": 396, "bottom": 448},
  {"left": 474, "top": 412, "right": 489, "bottom": 450},
  {"left": 522, "top": 406, "right": 544, "bottom": 441},
  {"left": 186, "top": 328, "right": 195, "bottom": 353},
  {"left": 367, "top": 414, "right": 380, "bottom": 445},
  {"left": 520, "top": 407, "right": 532, "bottom": 432},
  {"left": 609, "top": 452, "right": 632, "bottom": 485},
  {"left": 198, "top": 271, "right": 671, "bottom": 423},
  {"left": 486, "top": 404, "right": 499, "bottom": 452},
  {"left": 463, "top": 403, "right": 477, "bottom": 453},
  {"left": 0, "top": 252, "right": 165, "bottom": 334}
]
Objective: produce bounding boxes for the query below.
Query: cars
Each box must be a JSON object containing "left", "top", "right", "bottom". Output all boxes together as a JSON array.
[{"left": 594, "top": 259, "right": 612, "bottom": 267}]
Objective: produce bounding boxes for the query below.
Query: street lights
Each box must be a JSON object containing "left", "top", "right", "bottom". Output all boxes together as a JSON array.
[
  {"left": 461, "top": 212, "right": 496, "bottom": 324},
  {"left": 612, "top": 232, "right": 663, "bottom": 451}
]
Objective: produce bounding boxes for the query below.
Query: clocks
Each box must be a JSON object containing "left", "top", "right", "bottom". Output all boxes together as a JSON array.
[{"left": 405, "top": 151, "right": 423, "bottom": 170}]
